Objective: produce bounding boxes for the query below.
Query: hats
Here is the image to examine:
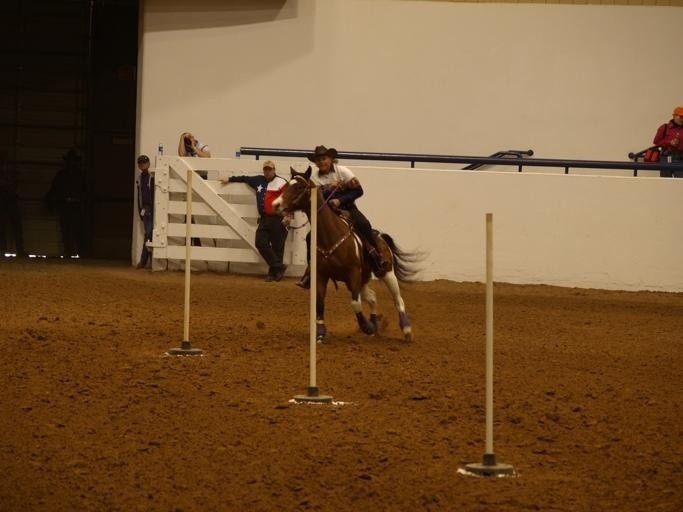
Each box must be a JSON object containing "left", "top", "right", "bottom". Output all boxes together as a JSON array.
[
  {"left": 307, "top": 144, "right": 337, "bottom": 162},
  {"left": 137, "top": 155, "right": 149, "bottom": 162},
  {"left": 672, "top": 106, "right": 683, "bottom": 116},
  {"left": 263, "top": 160, "right": 275, "bottom": 169}
]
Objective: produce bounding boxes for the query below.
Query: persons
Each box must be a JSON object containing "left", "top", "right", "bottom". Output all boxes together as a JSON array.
[
  {"left": 297, "top": 146, "right": 388, "bottom": 289},
  {"left": 45, "top": 151, "right": 95, "bottom": 260},
  {"left": 218, "top": 160, "right": 294, "bottom": 282},
  {"left": 0, "top": 151, "right": 28, "bottom": 259},
  {"left": 653, "top": 107, "right": 683, "bottom": 178},
  {"left": 136, "top": 155, "right": 154, "bottom": 269},
  {"left": 178, "top": 133, "right": 212, "bottom": 179}
]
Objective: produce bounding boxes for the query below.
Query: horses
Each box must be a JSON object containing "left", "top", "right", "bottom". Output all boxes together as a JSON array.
[{"left": 270, "top": 164, "right": 422, "bottom": 344}]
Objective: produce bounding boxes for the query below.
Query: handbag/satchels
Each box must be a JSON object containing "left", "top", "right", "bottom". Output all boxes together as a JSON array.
[{"left": 645, "top": 148, "right": 659, "bottom": 162}]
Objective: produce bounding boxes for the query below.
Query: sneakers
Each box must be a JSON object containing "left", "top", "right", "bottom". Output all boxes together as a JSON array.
[
  {"left": 295, "top": 278, "right": 310, "bottom": 289},
  {"left": 266, "top": 263, "right": 288, "bottom": 281}
]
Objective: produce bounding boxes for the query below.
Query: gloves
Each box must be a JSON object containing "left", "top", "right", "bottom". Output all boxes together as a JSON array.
[{"left": 329, "top": 198, "right": 340, "bottom": 208}]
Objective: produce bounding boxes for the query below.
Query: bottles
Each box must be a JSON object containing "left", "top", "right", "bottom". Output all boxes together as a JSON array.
[
  {"left": 159, "top": 143, "right": 163, "bottom": 156},
  {"left": 673, "top": 132, "right": 680, "bottom": 144}
]
